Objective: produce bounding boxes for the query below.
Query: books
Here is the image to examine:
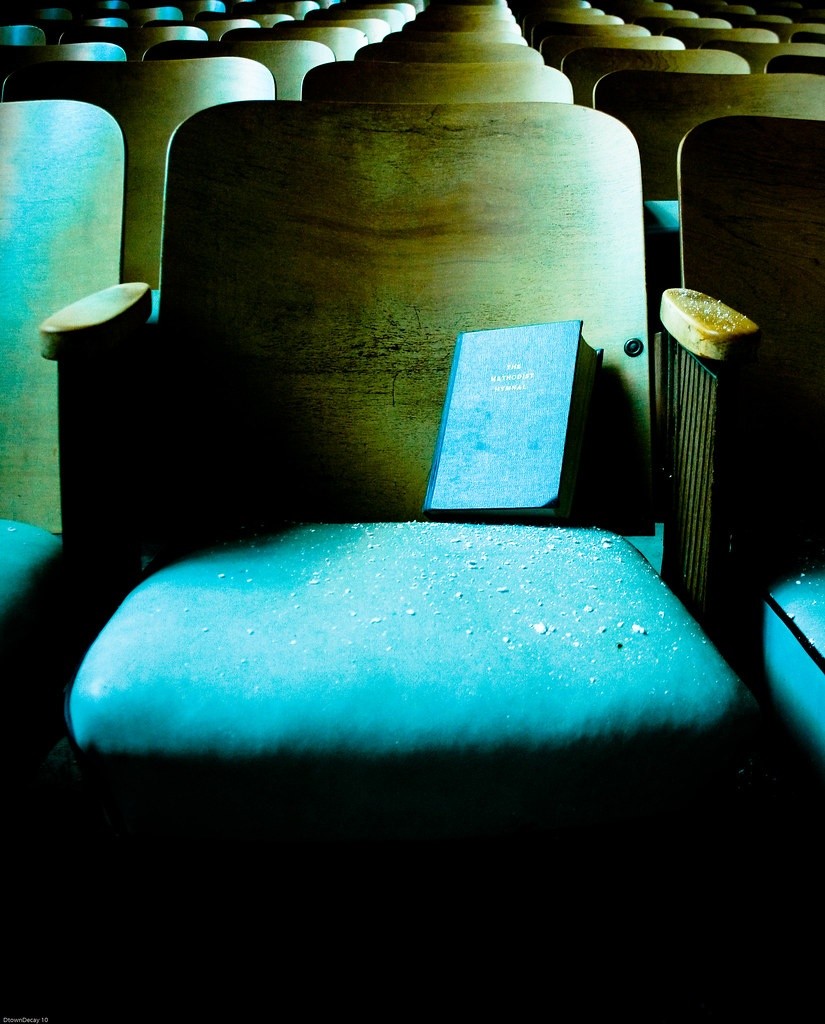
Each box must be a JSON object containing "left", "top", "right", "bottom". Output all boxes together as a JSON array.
[{"left": 421, "top": 320, "right": 605, "bottom": 526}]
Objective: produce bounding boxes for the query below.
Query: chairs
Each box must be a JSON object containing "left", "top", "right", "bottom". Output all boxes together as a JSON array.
[{"left": 0, "top": 0, "right": 825, "bottom": 763}]
